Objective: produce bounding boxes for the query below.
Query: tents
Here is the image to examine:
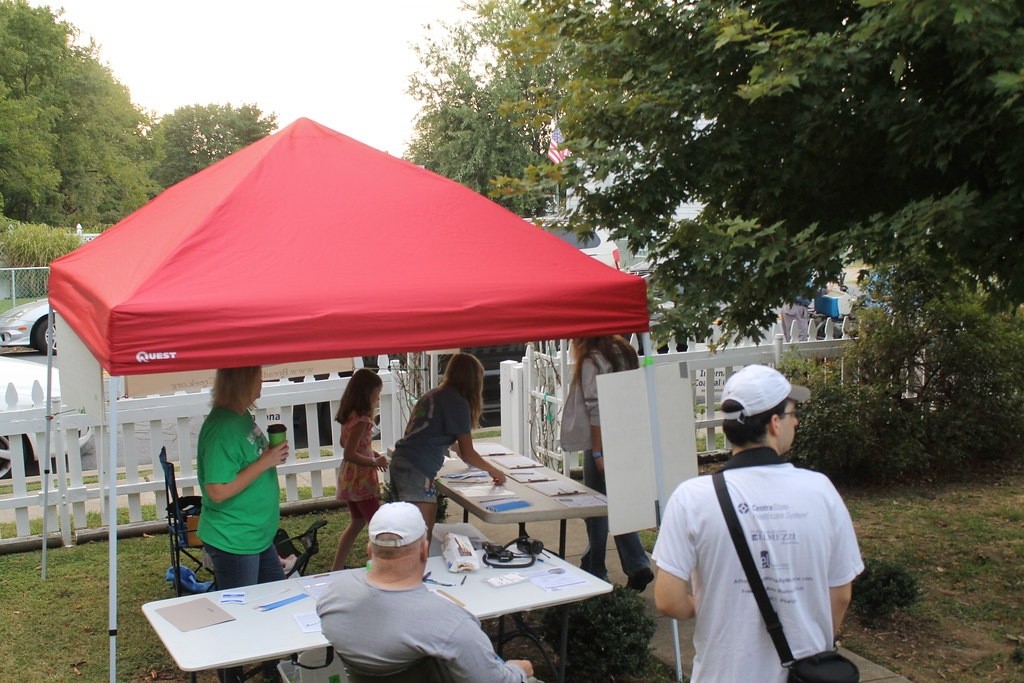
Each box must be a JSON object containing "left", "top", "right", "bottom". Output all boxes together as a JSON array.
[{"left": 40, "top": 116, "right": 683, "bottom": 683}]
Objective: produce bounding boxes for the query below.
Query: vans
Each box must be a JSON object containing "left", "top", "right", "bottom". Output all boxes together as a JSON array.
[{"left": 514, "top": 213, "right": 621, "bottom": 283}]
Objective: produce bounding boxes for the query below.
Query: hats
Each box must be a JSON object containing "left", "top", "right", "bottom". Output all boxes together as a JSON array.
[
  {"left": 368, "top": 501, "right": 428, "bottom": 548},
  {"left": 720, "top": 364, "right": 810, "bottom": 425}
]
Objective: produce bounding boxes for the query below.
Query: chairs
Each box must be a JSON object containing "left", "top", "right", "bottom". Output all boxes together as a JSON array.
[
  {"left": 156, "top": 444, "right": 217, "bottom": 597},
  {"left": 276, "top": 516, "right": 331, "bottom": 578}
]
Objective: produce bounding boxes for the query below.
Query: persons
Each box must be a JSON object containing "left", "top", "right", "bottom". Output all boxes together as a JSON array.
[
  {"left": 315, "top": 500, "right": 534, "bottom": 683},
  {"left": 183, "top": 365, "right": 290, "bottom": 683},
  {"left": 326, "top": 368, "right": 388, "bottom": 572},
  {"left": 560, "top": 333, "right": 655, "bottom": 594},
  {"left": 389, "top": 351, "right": 507, "bottom": 558},
  {"left": 652, "top": 363, "right": 866, "bottom": 683}
]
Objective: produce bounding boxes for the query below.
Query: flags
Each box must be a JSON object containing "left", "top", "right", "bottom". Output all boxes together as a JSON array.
[{"left": 547, "top": 126, "right": 572, "bottom": 165}]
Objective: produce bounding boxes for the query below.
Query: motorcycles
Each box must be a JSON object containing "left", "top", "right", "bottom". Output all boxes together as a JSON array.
[{"left": 810, "top": 271, "right": 915, "bottom": 338}]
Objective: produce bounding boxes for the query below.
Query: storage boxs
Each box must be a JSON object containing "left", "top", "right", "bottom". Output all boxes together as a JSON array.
[
  {"left": 276, "top": 658, "right": 350, "bottom": 683},
  {"left": 813, "top": 289, "right": 850, "bottom": 316}
]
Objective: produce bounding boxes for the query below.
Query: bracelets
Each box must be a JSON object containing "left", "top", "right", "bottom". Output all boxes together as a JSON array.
[
  {"left": 592, "top": 451, "right": 602, "bottom": 457},
  {"left": 371, "top": 458, "right": 376, "bottom": 467}
]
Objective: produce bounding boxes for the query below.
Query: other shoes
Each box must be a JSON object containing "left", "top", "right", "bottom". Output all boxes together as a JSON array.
[{"left": 624, "top": 572, "right": 654, "bottom": 594}]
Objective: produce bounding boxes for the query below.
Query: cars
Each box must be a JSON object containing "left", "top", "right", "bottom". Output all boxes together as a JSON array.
[
  {"left": 626, "top": 252, "right": 681, "bottom": 298},
  {"left": 0, "top": 295, "right": 123, "bottom": 354},
  {"left": 0, "top": 356, "right": 90, "bottom": 482}
]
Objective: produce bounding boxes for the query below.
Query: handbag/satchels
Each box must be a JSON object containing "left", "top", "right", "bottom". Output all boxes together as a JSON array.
[{"left": 787, "top": 649, "right": 860, "bottom": 683}]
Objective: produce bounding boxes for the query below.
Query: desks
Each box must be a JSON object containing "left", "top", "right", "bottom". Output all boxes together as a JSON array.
[
  {"left": 139, "top": 539, "right": 613, "bottom": 683},
  {"left": 385, "top": 439, "right": 609, "bottom": 641}
]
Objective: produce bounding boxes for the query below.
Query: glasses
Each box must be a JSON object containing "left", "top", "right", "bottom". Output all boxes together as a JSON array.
[{"left": 781, "top": 406, "right": 803, "bottom": 418}]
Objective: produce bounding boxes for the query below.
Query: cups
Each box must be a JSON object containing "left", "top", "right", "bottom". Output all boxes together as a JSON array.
[{"left": 267, "top": 424, "right": 287, "bottom": 464}]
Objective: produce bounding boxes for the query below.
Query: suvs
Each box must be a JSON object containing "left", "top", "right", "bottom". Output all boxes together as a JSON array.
[{"left": 275, "top": 331, "right": 573, "bottom": 444}]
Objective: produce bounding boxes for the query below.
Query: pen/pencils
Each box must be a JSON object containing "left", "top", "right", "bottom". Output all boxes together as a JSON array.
[
  {"left": 537, "top": 557, "right": 559, "bottom": 568},
  {"left": 422, "top": 572, "right": 432, "bottom": 581},
  {"left": 461, "top": 575, "right": 466, "bottom": 586},
  {"left": 510, "top": 472, "right": 535, "bottom": 475}
]
